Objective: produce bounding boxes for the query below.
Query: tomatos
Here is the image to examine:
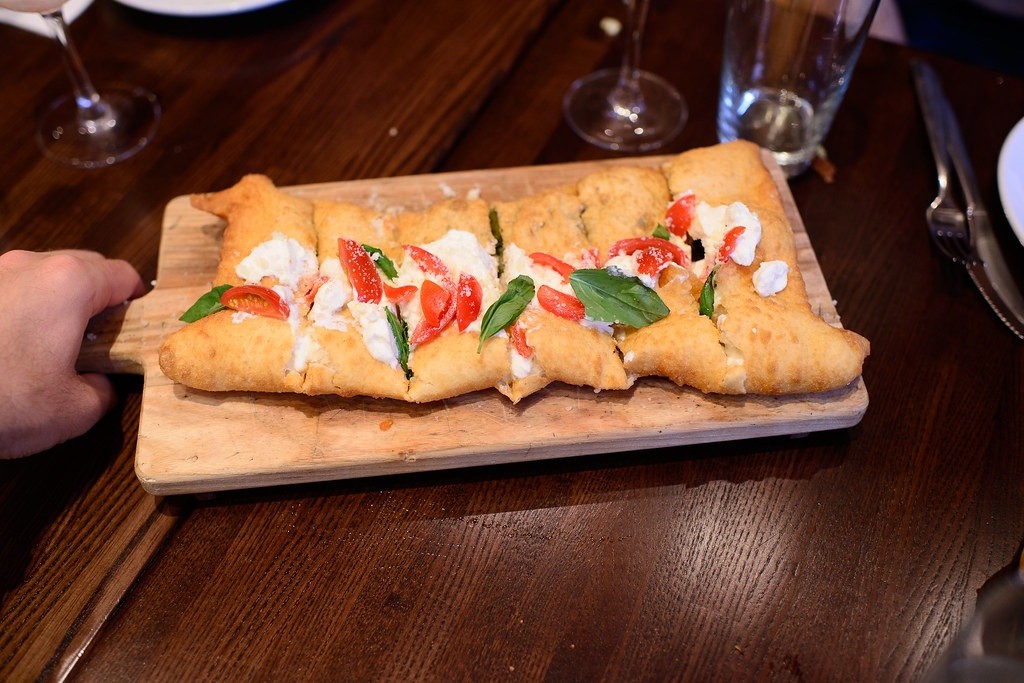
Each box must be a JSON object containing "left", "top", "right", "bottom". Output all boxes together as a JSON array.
[
  {"left": 221, "top": 286, "right": 290, "bottom": 319},
  {"left": 337, "top": 237, "right": 383, "bottom": 305},
  {"left": 383, "top": 193, "right": 745, "bottom": 358}
]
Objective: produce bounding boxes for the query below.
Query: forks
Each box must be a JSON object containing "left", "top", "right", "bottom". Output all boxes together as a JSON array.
[{"left": 913, "top": 60, "right": 990, "bottom": 271}]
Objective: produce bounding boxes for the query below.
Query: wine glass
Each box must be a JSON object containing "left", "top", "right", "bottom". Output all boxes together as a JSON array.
[
  {"left": 0, "top": 0, "right": 166, "bottom": 171},
  {"left": 560, "top": 0, "right": 690, "bottom": 152}
]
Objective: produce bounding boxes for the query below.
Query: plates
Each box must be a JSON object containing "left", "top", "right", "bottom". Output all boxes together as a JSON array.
[{"left": 996, "top": 117, "right": 1024, "bottom": 249}]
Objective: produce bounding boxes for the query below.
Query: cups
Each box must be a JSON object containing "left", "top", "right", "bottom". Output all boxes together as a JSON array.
[{"left": 713, "top": 0, "right": 880, "bottom": 183}]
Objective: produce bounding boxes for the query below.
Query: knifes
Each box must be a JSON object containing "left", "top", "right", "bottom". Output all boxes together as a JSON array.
[{"left": 934, "top": 87, "right": 1023, "bottom": 342}]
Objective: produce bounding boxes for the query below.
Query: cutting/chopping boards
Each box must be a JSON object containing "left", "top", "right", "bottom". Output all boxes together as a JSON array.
[{"left": 68, "top": 148, "right": 870, "bottom": 496}]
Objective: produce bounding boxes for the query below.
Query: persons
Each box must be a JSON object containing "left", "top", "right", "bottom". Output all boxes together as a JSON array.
[{"left": 0, "top": 247, "right": 146, "bottom": 461}]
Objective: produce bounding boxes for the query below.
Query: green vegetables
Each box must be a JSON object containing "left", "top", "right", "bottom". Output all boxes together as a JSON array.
[
  {"left": 361, "top": 224, "right": 720, "bottom": 373},
  {"left": 178, "top": 284, "right": 235, "bottom": 322}
]
